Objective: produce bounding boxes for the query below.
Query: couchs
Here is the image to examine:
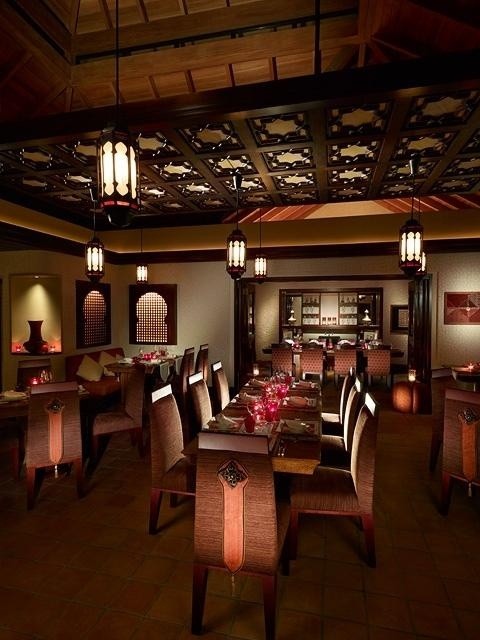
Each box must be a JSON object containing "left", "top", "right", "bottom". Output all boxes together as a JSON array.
[{"left": 64, "top": 347, "right": 125, "bottom": 396}]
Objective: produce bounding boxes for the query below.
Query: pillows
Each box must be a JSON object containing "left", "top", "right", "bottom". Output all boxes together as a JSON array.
[
  {"left": 115, "top": 353, "right": 124, "bottom": 360},
  {"left": 76, "top": 355, "right": 104, "bottom": 382},
  {"left": 98, "top": 351, "right": 117, "bottom": 377}
]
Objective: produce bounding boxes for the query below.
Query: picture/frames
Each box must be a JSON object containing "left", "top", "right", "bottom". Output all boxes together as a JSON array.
[
  {"left": 443, "top": 291, "right": 480, "bottom": 325},
  {"left": 390, "top": 305, "right": 410, "bottom": 334}
]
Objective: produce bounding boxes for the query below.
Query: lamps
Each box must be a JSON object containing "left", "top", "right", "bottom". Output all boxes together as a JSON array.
[
  {"left": 136, "top": 217, "right": 149, "bottom": 289},
  {"left": 84, "top": 186, "right": 105, "bottom": 285},
  {"left": 253, "top": 203, "right": 267, "bottom": 285},
  {"left": 226, "top": 174, "right": 247, "bottom": 280},
  {"left": 399, "top": 153, "right": 424, "bottom": 281},
  {"left": 96, "top": 0, "right": 142, "bottom": 230},
  {"left": 409, "top": 188, "right": 427, "bottom": 279}
]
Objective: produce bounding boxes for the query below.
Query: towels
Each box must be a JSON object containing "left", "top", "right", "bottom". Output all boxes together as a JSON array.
[
  {"left": 238, "top": 392, "right": 262, "bottom": 406},
  {"left": 217, "top": 412, "right": 241, "bottom": 431},
  {"left": 250, "top": 378, "right": 268, "bottom": 389}
]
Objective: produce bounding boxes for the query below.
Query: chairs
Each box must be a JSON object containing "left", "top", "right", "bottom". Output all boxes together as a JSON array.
[
  {"left": 23, "top": 381, "right": 84, "bottom": 512},
  {"left": 190, "top": 430, "right": 292, "bottom": 640},
  {"left": 0, "top": 418, "right": 19, "bottom": 486},
  {"left": 195, "top": 343, "right": 209, "bottom": 383},
  {"left": 16, "top": 358, "right": 53, "bottom": 454},
  {"left": 186, "top": 370, "right": 214, "bottom": 439},
  {"left": 211, "top": 360, "right": 231, "bottom": 416},
  {"left": 171, "top": 346, "right": 196, "bottom": 426},
  {"left": 148, "top": 383, "right": 198, "bottom": 536},
  {"left": 87, "top": 370, "right": 145, "bottom": 465}
]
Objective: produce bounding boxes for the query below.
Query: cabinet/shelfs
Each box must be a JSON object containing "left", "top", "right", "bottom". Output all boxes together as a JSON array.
[{"left": 279, "top": 287, "right": 384, "bottom": 343}]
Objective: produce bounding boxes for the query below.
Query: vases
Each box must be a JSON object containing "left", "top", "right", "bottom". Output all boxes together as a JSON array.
[
  {"left": 287, "top": 309, "right": 296, "bottom": 326},
  {"left": 361, "top": 309, "right": 371, "bottom": 326},
  {"left": 23, "top": 320, "right": 49, "bottom": 353}
]
